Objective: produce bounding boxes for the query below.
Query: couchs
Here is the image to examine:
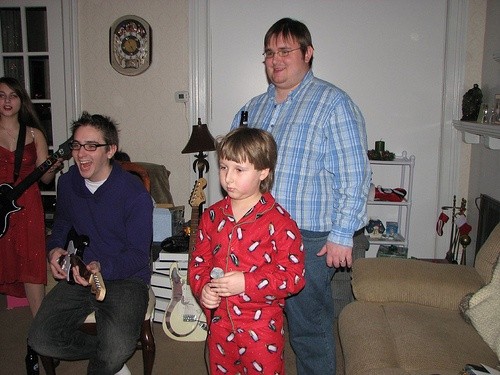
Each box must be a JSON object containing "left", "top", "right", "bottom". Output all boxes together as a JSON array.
[{"left": 337, "top": 221, "right": 500, "bottom": 375}]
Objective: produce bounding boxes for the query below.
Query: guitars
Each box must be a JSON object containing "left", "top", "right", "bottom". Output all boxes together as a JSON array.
[
  {"left": 57, "top": 225, "right": 108, "bottom": 302},
  {"left": 162, "top": 177, "right": 210, "bottom": 343},
  {"left": 0, "top": 134, "right": 75, "bottom": 239}
]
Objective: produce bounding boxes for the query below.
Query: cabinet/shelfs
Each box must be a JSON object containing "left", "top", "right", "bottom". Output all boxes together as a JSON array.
[{"left": 364, "top": 151, "right": 415, "bottom": 258}]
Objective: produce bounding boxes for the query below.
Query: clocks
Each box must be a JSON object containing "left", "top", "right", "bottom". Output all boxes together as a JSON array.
[{"left": 109, "top": 15, "right": 151, "bottom": 76}]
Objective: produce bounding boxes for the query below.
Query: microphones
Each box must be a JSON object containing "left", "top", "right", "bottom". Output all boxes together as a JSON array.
[{"left": 210, "top": 266, "right": 224, "bottom": 319}]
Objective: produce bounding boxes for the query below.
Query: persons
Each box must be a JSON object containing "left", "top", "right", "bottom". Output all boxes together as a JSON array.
[
  {"left": 28, "top": 111, "right": 157, "bottom": 375},
  {"left": 230, "top": 17, "right": 372, "bottom": 375},
  {"left": 0, "top": 76, "right": 66, "bottom": 375},
  {"left": 188, "top": 127, "right": 308, "bottom": 375}
]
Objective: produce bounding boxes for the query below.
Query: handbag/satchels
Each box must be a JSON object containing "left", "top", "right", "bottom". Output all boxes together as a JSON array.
[{"left": 374, "top": 185, "right": 408, "bottom": 202}]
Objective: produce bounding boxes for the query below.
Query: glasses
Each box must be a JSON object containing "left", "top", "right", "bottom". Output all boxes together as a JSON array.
[
  {"left": 262, "top": 47, "right": 301, "bottom": 58},
  {"left": 68, "top": 139, "right": 112, "bottom": 152}
]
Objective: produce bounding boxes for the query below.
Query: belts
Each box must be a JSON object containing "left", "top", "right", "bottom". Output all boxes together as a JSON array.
[{"left": 352, "top": 227, "right": 365, "bottom": 237}]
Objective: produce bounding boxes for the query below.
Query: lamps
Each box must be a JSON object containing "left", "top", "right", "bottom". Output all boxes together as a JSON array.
[{"left": 181, "top": 117, "right": 218, "bottom": 208}]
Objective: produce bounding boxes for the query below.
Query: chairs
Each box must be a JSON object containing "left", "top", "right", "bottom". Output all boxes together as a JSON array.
[{"left": 25, "top": 162, "right": 156, "bottom": 375}]
